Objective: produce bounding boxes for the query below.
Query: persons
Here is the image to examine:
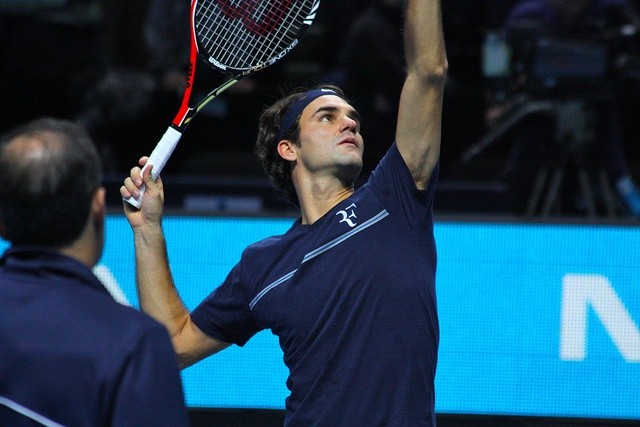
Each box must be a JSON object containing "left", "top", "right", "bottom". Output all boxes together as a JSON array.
[
  {"left": 2, "top": 118, "right": 188, "bottom": 427},
  {"left": 120, "top": 0, "right": 451, "bottom": 424}
]
